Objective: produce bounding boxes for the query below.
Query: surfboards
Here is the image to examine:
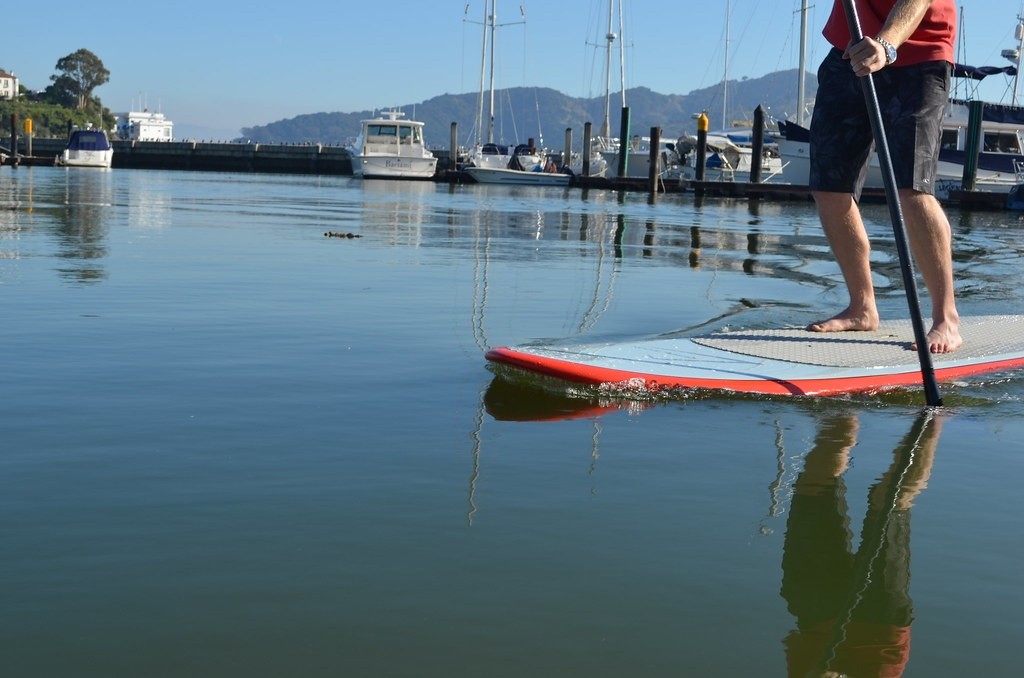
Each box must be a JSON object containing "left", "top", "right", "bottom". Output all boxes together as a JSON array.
[{"left": 483, "top": 316, "right": 1023, "bottom": 405}]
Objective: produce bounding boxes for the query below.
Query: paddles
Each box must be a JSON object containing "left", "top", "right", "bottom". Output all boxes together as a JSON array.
[{"left": 845, "top": 2, "right": 945, "bottom": 408}]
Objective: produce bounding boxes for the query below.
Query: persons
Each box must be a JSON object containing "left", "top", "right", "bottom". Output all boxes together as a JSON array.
[
  {"left": 781, "top": 403, "right": 949, "bottom": 678},
  {"left": 809, "top": 0, "right": 964, "bottom": 354}
]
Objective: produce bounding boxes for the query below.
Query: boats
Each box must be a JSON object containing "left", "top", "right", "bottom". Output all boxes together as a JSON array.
[
  {"left": 54, "top": 104, "right": 118, "bottom": 168},
  {"left": 348, "top": 100, "right": 438, "bottom": 179}
]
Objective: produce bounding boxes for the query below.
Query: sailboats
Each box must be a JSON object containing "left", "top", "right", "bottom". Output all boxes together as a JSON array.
[{"left": 459, "top": 1, "right": 1024, "bottom": 206}]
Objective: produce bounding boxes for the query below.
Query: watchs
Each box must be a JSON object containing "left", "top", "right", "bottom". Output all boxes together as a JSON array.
[{"left": 874, "top": 36, "right": 897, "bottom": 65}]
